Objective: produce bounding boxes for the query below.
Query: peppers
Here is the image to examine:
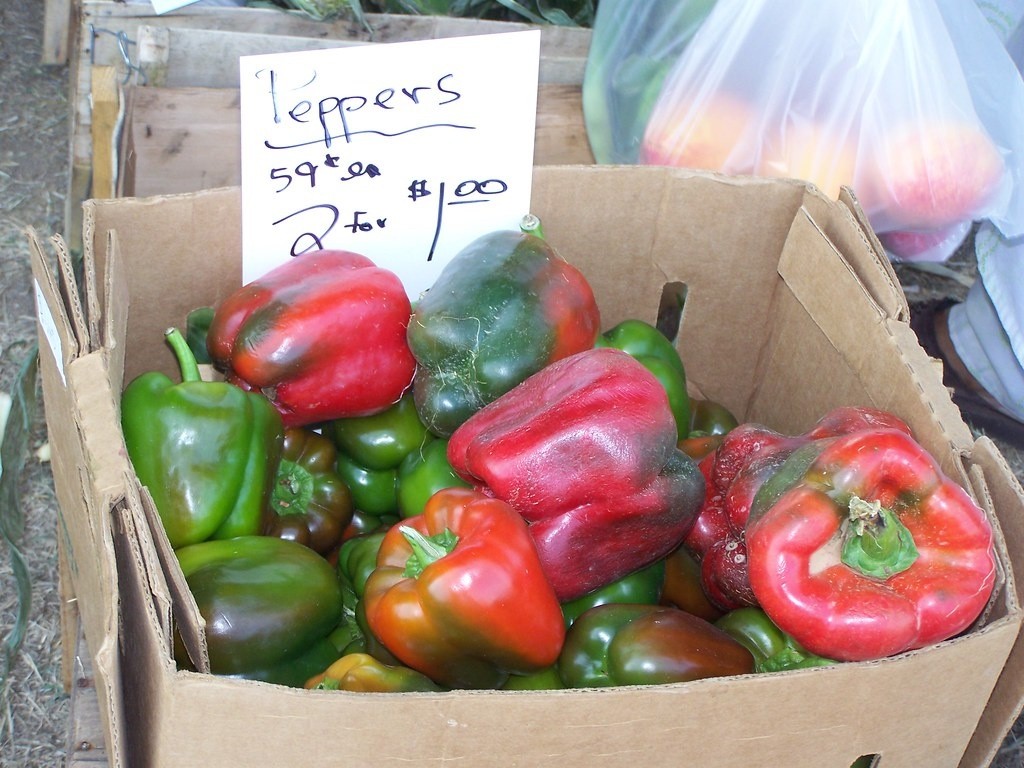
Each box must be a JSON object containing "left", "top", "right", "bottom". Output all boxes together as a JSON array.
[
  {"left": 118, "top": 216, "right": 998, "bottom": 700},
  {"left": 638, "top": 87, "right": 1000, "bottom": 259}
]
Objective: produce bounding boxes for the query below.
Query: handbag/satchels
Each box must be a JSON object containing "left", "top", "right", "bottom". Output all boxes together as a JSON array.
[{"left": 582, "top": 0, "right": 1024, "bottom": 264}]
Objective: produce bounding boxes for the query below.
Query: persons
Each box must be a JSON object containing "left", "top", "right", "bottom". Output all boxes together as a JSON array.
[{"left": 908, "top": 0, "right": 1024, "bottom": 440}]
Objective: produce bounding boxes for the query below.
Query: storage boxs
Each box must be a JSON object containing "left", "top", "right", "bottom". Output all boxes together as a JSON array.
[{"left": 15, "top": 0, "right": 1024, "bottom": 768}]
